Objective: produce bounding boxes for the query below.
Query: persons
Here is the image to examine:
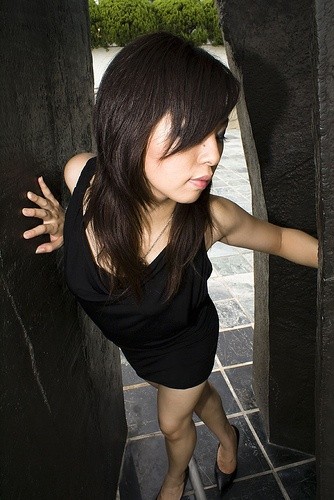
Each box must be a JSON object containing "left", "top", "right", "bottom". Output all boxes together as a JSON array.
[{"left": 23, "top": 31, "right": 321, "bottom": 500}]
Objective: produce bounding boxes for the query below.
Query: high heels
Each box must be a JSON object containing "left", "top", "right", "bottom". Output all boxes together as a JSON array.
[
  {"left": 214, "top": 424, "right": 240, "bottom": 497},
  {"left": 155, "top": 465, "right": 189, "bottom": 500}
]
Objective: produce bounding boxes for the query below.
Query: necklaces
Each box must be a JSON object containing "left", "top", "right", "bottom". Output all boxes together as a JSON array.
[{"left": 116, "top": 215, "right": 172, "bottom": 267}]
